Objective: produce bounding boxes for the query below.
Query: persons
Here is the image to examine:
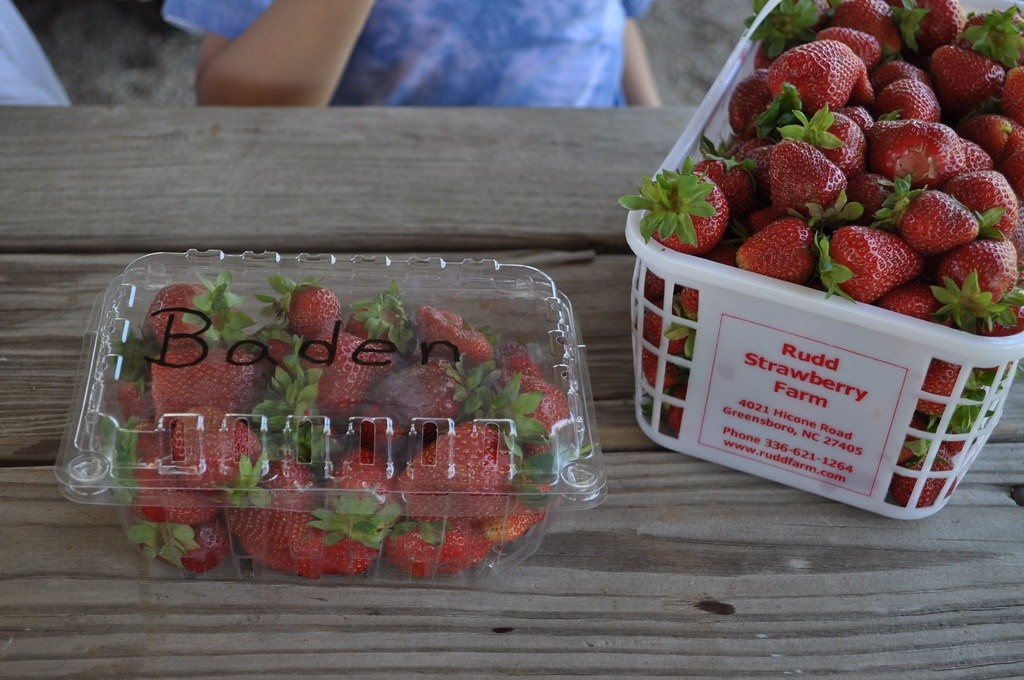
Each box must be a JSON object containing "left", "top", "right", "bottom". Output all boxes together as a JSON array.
[{"left": 160, "top": 0, "right": 662, "bottom": 107}]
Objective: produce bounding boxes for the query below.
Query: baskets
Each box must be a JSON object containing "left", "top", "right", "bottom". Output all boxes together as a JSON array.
[{"left": 624, "top": 0, "right": 1023, "bottom": 521}]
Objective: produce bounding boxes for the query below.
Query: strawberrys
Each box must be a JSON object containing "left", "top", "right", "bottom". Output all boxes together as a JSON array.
[
  {"left": 619, "top": 0, "right": 1024, "bottom": 508},
  {"left": 100, "top": 269, "right": 571, "bottom": 578}
]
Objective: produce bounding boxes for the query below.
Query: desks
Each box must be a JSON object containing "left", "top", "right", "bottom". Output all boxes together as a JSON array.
[{"left": 0, "top": 105, "right": 1023, "bottom": 680}]
